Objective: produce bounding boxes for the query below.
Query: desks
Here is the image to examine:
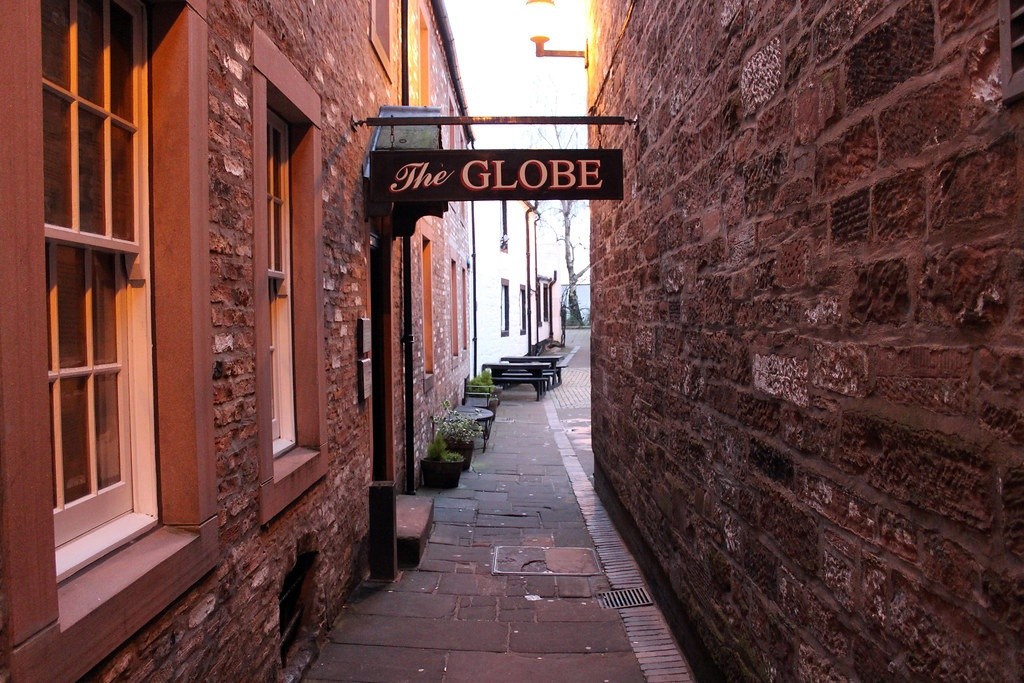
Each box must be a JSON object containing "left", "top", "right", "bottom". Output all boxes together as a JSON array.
[
  {"left": 431, "top": 405, "right": 493, "bottom": 452},
  {"left": 482, "top": 363, "right": 551, "bottom": 394},
  {"left": 501, "top": 355, "right": 562, "bottom": 386}
]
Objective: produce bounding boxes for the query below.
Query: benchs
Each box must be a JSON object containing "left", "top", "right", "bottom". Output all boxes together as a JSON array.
[
  {"left": 502, "top": 372, "right": 554, "bottom": 391},
  {"left": 491, "top": 378, "right": 549, "bottom": 400},
  {"left": 551, "top": 365, "right": 568, "bottom": 382},
  {"left": 507, "top": 368, "right": 559, "bottom": 386}
]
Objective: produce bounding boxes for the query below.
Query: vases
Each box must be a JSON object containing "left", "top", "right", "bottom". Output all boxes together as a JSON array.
[{"left": 444, "top": 440, "right": 474, "bottom": 471}]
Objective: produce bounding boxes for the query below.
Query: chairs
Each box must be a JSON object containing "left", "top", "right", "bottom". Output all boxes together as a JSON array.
[{"left": 464, "top": 374, "right": 492, "bottom": 423}]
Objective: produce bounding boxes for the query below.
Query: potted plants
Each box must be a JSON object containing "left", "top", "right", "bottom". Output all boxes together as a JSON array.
[
  {"left": 476, "top": 369, "right": 502, "bottom": 404},
  {"left": 420, "top": 429, "right": 465, "bottom": 488},
  {"left": 467, "top": 376, "right": 499, "bottom": 422}
]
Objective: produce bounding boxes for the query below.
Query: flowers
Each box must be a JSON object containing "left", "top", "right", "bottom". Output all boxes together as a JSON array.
[{"left": 430, "top": 399, "right": 485, "bottom": 443}]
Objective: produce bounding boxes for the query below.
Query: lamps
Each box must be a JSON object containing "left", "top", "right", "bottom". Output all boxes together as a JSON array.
[{"left": 525, "top": 0, "right": 590, "bottom": 68}]
